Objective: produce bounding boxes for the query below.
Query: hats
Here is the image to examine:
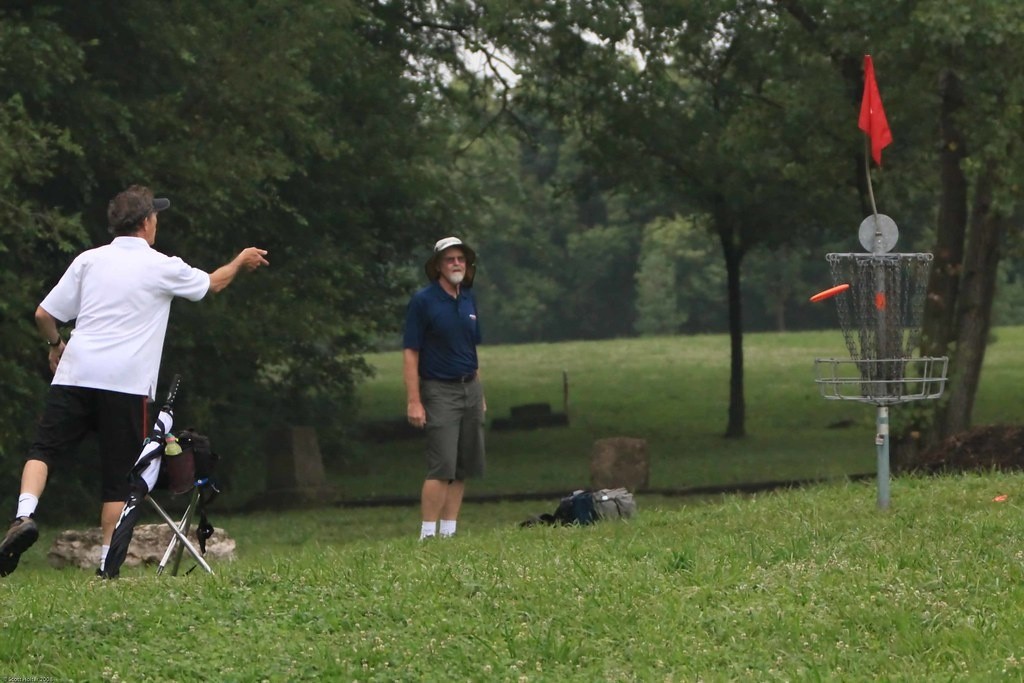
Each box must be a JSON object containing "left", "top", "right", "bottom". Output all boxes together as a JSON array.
[
  {"left": 108, "top": 184, "right": 170, "bottom": 223},
  {"left": 425, "top": 236, "right": 476, "bottom": 285}
]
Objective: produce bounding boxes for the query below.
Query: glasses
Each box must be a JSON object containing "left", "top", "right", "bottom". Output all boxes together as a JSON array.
[{"left": 437, "top": 255, "right": 466, "bottom": 263}]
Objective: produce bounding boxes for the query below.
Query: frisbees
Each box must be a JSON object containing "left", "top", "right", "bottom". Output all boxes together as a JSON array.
[{"left": 810, "top": 283, "right": 850, "bottom": 303}]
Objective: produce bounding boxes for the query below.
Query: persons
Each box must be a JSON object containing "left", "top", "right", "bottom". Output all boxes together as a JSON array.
[
  {"left": 0, "top": 184, "right": 270, "bottom": 579},
  {"left": 401, "top": 237, "right": 486, "bottom": 547}
]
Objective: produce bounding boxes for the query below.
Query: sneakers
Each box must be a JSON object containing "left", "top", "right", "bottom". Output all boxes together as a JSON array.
[{"left": 0, "top": 513, "right": 38, "bottom": 578}]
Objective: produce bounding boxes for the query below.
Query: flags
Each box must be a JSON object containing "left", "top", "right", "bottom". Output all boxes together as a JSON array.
[{"left": 858, "top": 55, "right": 893, "bottom": 165}]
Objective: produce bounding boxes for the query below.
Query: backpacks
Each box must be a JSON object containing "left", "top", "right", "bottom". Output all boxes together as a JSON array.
[{"left": 550, "top": 486, "right": 636, "bottom": 527}]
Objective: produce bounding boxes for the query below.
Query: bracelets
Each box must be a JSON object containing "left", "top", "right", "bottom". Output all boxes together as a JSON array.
[{"left": 46, "top": 334, "right": 61, "bottom": 346}]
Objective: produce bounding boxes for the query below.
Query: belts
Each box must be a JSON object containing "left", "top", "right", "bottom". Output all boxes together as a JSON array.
[{"left": 423, "top": 373, "right": 477, "bottom": 384}]
